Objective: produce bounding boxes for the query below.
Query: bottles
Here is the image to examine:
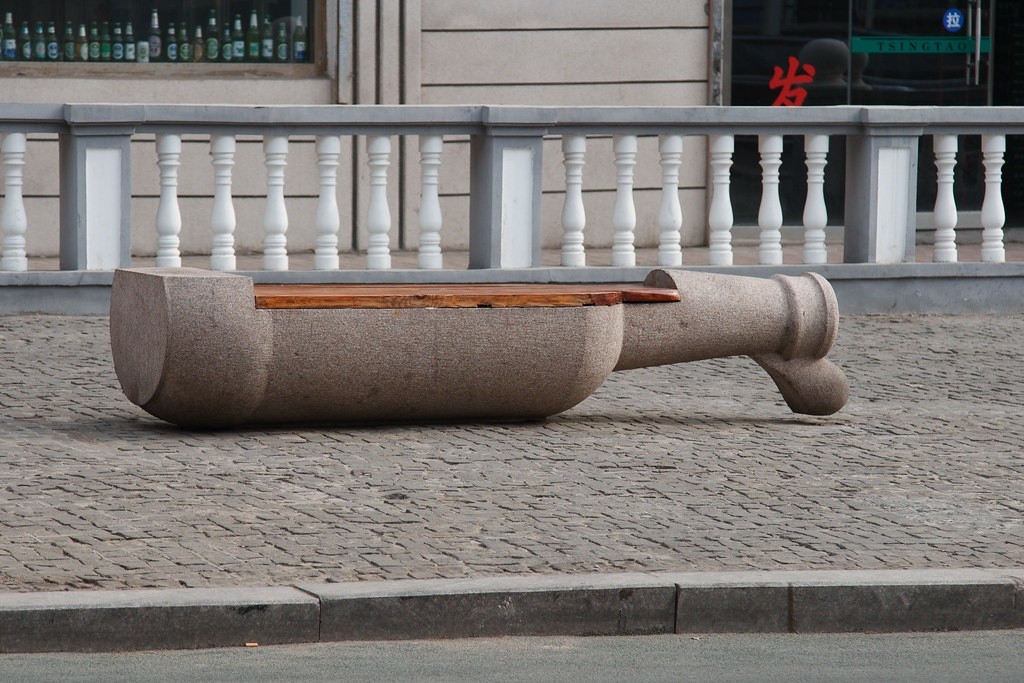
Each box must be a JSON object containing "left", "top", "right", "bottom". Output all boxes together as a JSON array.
[{"left": 1, "top": 8, "right": 308, "bottom": 63}]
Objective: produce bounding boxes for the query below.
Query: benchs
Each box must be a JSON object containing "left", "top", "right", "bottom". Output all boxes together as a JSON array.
[{"left": 108, "top": 266, "right": 850, "bottom": 431}]
